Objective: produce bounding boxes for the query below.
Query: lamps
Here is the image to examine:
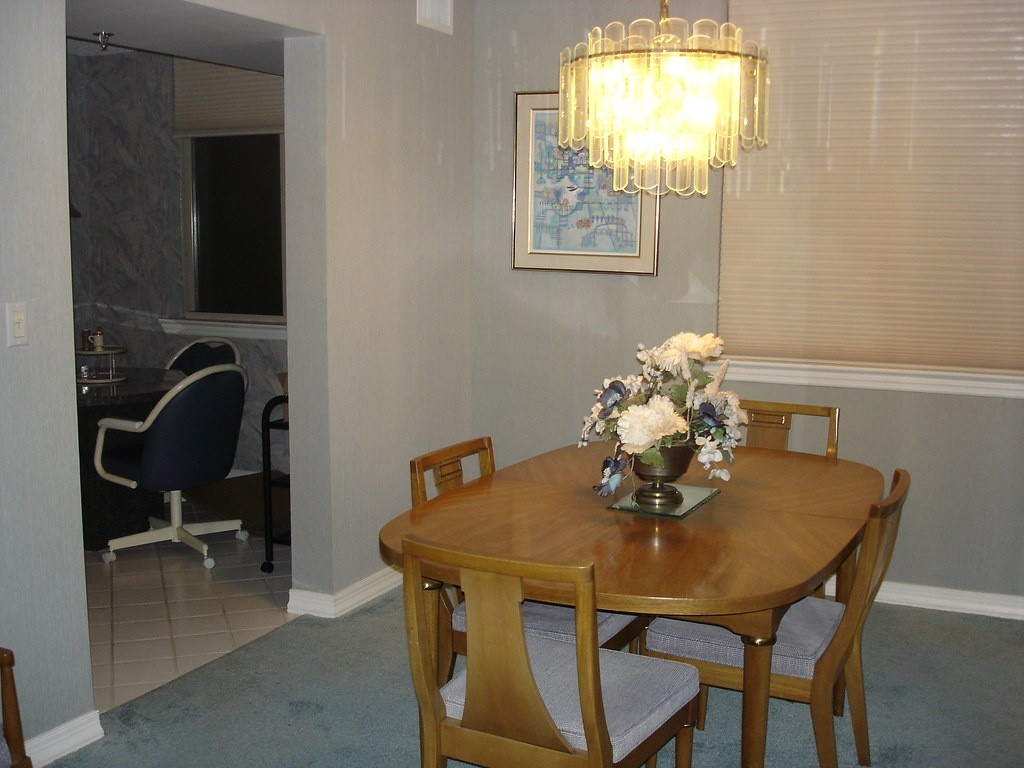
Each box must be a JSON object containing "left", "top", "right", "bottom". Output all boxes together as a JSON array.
[{"left": 558, "top": 0, "right": 769, "bottom": 199}]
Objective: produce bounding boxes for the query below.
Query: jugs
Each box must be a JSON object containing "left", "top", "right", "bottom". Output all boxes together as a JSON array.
[{"left": 88, "top": 330, "right": 104, "bottom": 351}]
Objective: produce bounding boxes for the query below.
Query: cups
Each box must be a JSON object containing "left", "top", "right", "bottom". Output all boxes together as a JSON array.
[
  {"left": 82, "top": 329, "right": 93, "bottom": 351},
  {"left": 81, "top": 365, "right": 89, "bottom": 378}
]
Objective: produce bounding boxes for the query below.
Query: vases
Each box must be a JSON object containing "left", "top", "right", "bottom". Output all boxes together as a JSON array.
[{"left": 625, "top": 442, "right": 699, "bottom": 505}]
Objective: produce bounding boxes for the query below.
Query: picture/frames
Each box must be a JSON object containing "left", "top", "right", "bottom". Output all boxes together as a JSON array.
[{"left": 512, "top": 91, "right": 658, "bottom": 276}]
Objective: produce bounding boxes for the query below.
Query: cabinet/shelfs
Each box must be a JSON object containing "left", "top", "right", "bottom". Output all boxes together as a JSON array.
[{"left": 260, "top": 393, "right": 290, "bottom": 573}]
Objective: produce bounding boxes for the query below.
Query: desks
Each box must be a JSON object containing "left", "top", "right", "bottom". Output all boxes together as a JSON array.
[
  {"left": 378, "top": 436, "right": 885, "bottom": 768},
  {"left": 76, "top": 368, "right": 187, "bottom": 406}
]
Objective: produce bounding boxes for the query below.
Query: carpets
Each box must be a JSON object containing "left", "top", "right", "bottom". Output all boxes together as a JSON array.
[{"left": 175, "top": 468, "right": 292, "bottom": 545}]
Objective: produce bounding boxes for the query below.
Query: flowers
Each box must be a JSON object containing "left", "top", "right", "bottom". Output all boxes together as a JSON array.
[{"left": 577, "top": 332, "right": 748, "bottom": 507}]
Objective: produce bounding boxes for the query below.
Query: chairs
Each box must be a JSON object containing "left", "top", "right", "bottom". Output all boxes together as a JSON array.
[
  {"left": 165, "top": 335, "right": 241, "bottom": 376},
  {"left": 94, "top": 365, "right": 252, "bottom": 568},
  {"left": 410, "top": 436, "right": 648, "bottom": 692},
  {"left": 638, "top": 466, "right": 915, "bottom": 768},
  {"left": 736, "top": 396, "right": 842, "bottom": 601},
  {"left": 397, "top": 532, "right": 698, "bottom": 767}
]
